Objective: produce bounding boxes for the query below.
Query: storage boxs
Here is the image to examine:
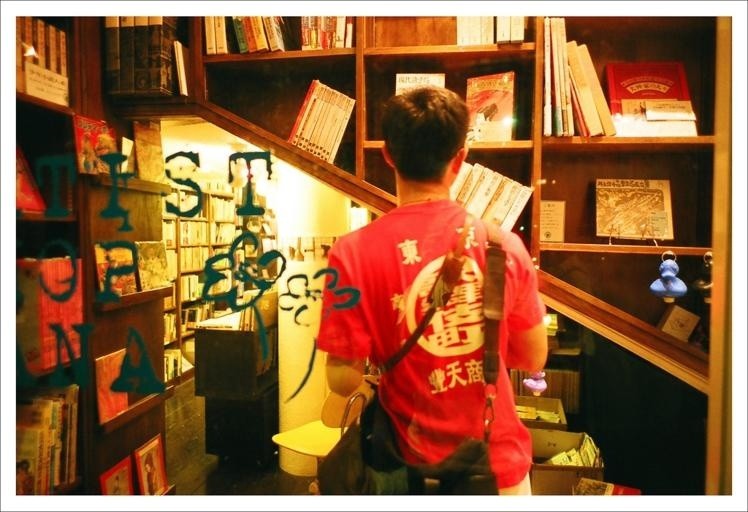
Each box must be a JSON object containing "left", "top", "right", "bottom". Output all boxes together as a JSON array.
[
  {"left": 529, "top": 426, "right": 605, "bottom": 495},
  {"left": 514, "top": 392, "right": 568, "bottom": 431}
]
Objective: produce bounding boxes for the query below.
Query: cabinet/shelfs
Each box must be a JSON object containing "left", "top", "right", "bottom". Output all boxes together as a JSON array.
[
  {"left": 15, "top": 15, "right": 91, "bottom": 496},
  {"left": 100, "top": 15, "right": 194, "bottom": 110},
  {"left": 84, "top": 173, "right": 177, "bottom": 498},
  {"left": 358, "top": 16, "right": 536, "bottom": 267},
  {"left": 195, "top": 15, "right": 358, "bottom": 185},
  {"left": 163, "top": 185, "right": 180, "bottom": 392},
  {"left": 208, "top": 191, "right": 235, "bottom": 317},
  {"left": 535, "top": 16, "right": 732, "bottom": 261},
  {"left": 178, "top": 185, "right": 209, "bottom": 384}
]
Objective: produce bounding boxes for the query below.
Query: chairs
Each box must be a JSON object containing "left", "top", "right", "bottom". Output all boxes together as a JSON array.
[{"left": 271, "top": 375, "right": 376, "bottom": 496}]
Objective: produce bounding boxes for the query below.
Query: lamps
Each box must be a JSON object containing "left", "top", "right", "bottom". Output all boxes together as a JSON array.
[{"left": 276, "top": 177, "right": 349, "bottom": 263}]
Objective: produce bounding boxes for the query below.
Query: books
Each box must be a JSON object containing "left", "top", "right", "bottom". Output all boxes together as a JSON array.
[
  {"left": 127, "top": 118, "right": 165, "bottom": 183},
  {"left": 14, "top": 16, "right": 71, "bottom": 107},
  {"left": 202, "top": 16, "right": 356, "bottom": 57},
  {"left": 164, "top": 159, "right": 267, "bottom": 386},
  {"left": 101, "top": 17, "right": 192, "bottom": 99},
  {"left": 135, "top": 432, "right": 168, "bottom": 496},
  {"left": 507, "top": 313, "right": 592, "bottom": 420},
  {"left": 366, "top": 18, "right": 528, "bottom": 47},
  {"left": 544, "top": 17, "right": 700, "bottom": 138},
  {"left": 95, "top": 241, "right": 137, "bottom": 298},
  {"left": 72, "top": 115, "right": 120, "bottom": 174},
  {"left": 464, "top": 71, "right": 516, "bottom": 143},
  {"left": 133, "top": 239, "right": 173, "bottom": 293},
  {"left": 16, "top": 381, "right": 78, "bottom": 495},
  {"left": 17, "top": 250, "right": 83, "bottom": 374},
  {"left": 288, "top": 78, "right": 356, "bottom": 164},
  {"left": 92, "top": 348, "right": 131, "bottom": 424},
  {"left": 448, "top": 160, "right": 533, "bottom": 235},
  {"left": 394, "top": 72, "right": 447, "bottom": 102},
  {"left": 99, "top": 455, "right": 132, "bottom": 496}
]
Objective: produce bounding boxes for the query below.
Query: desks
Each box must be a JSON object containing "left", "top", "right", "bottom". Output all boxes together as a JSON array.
[{"left": 186, "top": 286, "right": 279, "bottom": 459}]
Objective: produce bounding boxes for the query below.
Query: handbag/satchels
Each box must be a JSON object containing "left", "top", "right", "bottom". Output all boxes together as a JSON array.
[{"left": 315, "top": 221, "right": 504, "bottom": 496}]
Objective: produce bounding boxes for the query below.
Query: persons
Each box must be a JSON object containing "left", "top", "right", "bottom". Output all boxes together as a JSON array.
[{"left": 313, "top": 85, "right": 548, "bottom": 496}]
